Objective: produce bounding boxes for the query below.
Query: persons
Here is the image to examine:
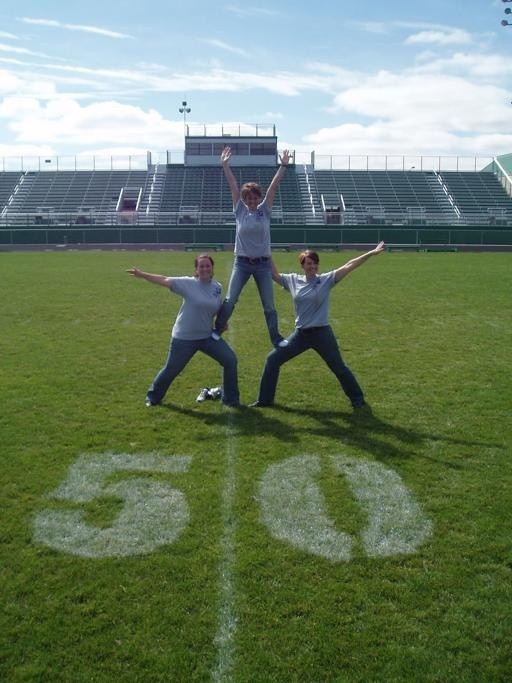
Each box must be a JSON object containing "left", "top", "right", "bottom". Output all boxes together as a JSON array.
[
  {"left": 211, "top": 143, "right": 291, "bottom": 349},
  {"left": 247, "top": 239, "right": 387, "bottom": 407},
  {"left": 125, "top": 252, "right": 246, "bottom": 410}
]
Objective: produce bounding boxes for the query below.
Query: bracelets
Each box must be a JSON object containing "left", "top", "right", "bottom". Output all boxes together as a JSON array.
[{"left": 280, "top": 163, "right": 288, "bottom": 168}]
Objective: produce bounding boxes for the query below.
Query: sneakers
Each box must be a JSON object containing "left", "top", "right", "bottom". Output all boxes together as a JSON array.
[
  {"left": 349, "top": 403, "right": 372, "bottom": 420},
  {"left": 197, "top": 385, "right": 222, "bottom": 403},
  {"left": 247, "top": 400, "right": 274, "bottom": 407}
]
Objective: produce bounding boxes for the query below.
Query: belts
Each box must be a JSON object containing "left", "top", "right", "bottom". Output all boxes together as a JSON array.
[{"left": 236, "top": 254, "right": 271, "bottom": 263}]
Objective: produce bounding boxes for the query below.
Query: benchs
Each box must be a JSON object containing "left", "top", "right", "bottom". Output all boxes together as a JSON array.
[{"left": 0, "top": 170, "right": 512, "bottom": 224}]
